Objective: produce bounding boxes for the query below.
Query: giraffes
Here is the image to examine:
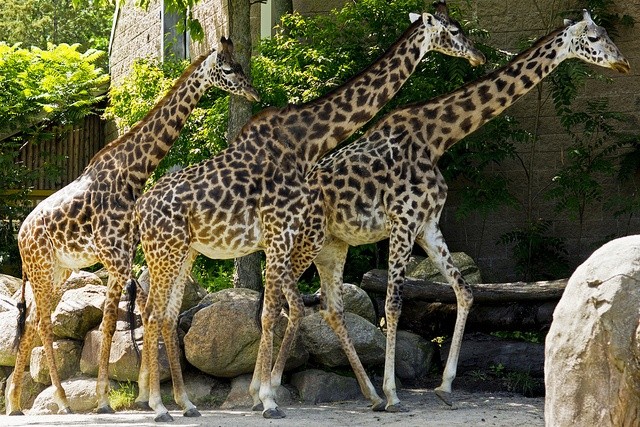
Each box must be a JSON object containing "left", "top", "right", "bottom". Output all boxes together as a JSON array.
[
  {"left": 248, "top": 8, "right": 631, "bottom": 413},
  {"left": 6, "top": 35, "right": 262, "bottom": 417},
  {"left": 124, "top": 0, "right": 487, "bottom": 423}
]
